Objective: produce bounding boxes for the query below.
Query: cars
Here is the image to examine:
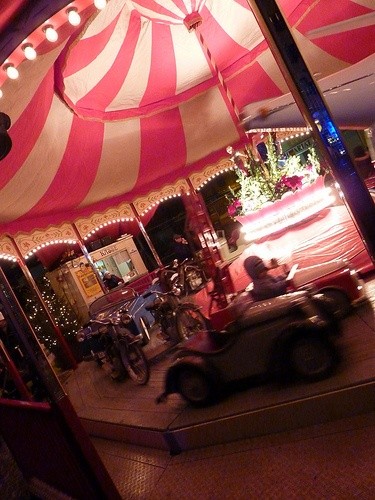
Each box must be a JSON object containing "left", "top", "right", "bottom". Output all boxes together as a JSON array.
[{"left": 159, "top": 254, "right": 370, "bottom": 410}]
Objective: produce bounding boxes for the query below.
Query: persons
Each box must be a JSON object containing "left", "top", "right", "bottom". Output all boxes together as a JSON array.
[
  {"left": 172, "top": 234, "right": 193, "bottom": 262},
  {"left": 245, "top": 256, "right": 290, "bottom": 300},
  {"left": 104, "top": 271, "right": 127, "bottom": 290}
]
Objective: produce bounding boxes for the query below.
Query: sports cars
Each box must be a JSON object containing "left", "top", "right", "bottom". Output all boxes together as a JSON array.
[{"left": 75, "top": 282, "right": 164, "bottom": 362}]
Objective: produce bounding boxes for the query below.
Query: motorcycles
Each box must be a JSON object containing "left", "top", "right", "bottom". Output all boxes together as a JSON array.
[
  {"left": 140, "top": 278, "right": 210, "bottom": 351},
  {"left": 81, "top": 307, "right": 152, "bottom": 387}
]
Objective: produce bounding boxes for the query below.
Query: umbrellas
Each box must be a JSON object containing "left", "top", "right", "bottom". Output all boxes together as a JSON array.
[{"left": 0, "top": 0, "right": 375, "bottom": 266}]
